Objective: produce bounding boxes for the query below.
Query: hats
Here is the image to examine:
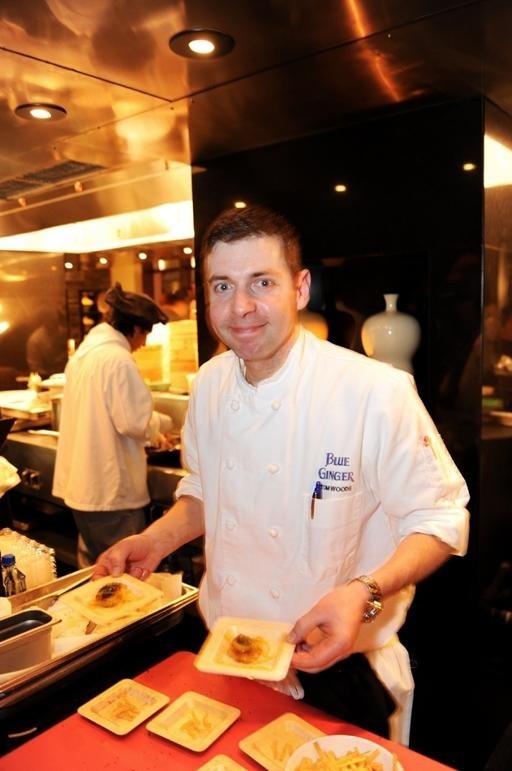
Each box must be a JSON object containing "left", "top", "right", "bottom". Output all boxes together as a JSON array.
[{"left": 103, "top": 282, "right": 170, "bottom": 326}]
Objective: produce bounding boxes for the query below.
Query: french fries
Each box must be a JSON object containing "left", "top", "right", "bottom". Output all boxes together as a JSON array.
[{"left": 291, "top": 740, "right": 399, "bottom": 771}]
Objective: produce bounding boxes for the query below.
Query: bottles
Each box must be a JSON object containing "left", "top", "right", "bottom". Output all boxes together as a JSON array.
[
  {"left": 0, "top": 554, "right": 27, "bottom": 597},
  {"left": 361, "top": 294, "right": 424, "bottom": 374}
]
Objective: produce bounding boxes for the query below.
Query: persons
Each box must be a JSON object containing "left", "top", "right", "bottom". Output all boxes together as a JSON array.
[
  {"left": 92, "top": 205, "right": 473, "bottom": 746},
  {"left": 51, "top": 282, "right": 170, "bottom": 562}
]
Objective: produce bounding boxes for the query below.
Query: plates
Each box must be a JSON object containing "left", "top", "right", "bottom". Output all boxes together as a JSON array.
[
  {"left": 76, "top": 679, "right": 242, "bottom": 753},
  {"left": 191, "top": 711, "right": 405, "bottom": 771},
  {"left": 61, "top": 572, "right": 166, "bottom": 625},
  {"left": 482, "top": 385, "right": 511, "bottom": 428},
  {"left": 192, "top": 617, "right": 303, "bottom": 682}
]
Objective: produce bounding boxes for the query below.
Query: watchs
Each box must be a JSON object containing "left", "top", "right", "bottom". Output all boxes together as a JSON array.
[{"left": 350, "top": 574, "right": 385, "bottom": 625}]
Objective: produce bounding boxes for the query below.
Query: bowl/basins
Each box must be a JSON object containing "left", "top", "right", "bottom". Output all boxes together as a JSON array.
[{"left": 153, "top": 383, "right": 174, "bottom": 391}]
